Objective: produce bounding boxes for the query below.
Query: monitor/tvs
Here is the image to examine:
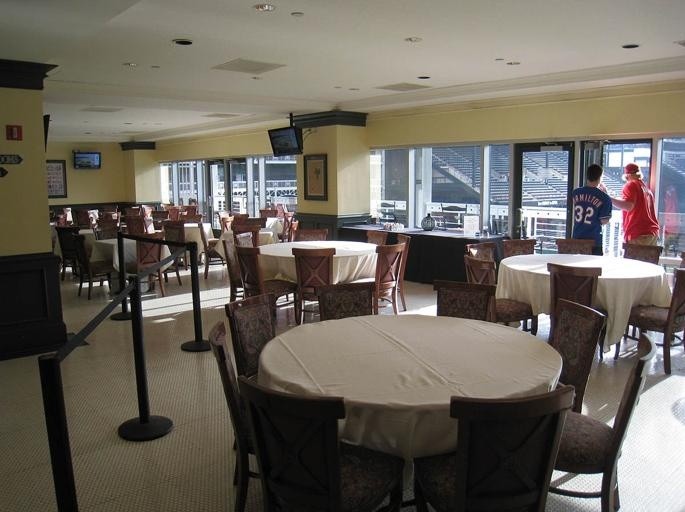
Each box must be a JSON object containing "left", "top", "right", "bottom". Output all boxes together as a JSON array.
[
  {"left": 268, "top": 127, "right": 303, "bottom": 157},
  {"left": 74, "top": 152, "right": 101, "bottom": 169},
  {"left": 43, "top": 114, "right": 50, "bottom": 153}
]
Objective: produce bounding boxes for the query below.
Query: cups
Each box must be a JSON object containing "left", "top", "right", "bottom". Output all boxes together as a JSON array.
[{"left": 382, "top": 222, "right": 404, "bottom": 232}]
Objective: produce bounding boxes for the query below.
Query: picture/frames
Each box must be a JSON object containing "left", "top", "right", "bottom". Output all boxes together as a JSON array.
[
  {"left": 46, "top": 159, "right": 67, "bottom": 199},
  {"left": 303, "top": 152, "right": 328, "bottom": 200}
]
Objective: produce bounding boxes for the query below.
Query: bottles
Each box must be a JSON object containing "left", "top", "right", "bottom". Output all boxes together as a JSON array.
[
  {"left": 481, "top": 225, "right": 489, "bottom": 238},
  {"left": 495, "top": 215, "right": 502, "bottom": 235},
  {"left": 420, "top": 212, "right": 435, "bottom": 231},
  {"left": 490, "top": 214, "right": 497, "bottom": 235}
]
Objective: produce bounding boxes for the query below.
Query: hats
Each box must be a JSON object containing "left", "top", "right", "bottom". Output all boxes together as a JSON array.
[{"left": 624, "top": 163, "right": 640, "bottom": 174}]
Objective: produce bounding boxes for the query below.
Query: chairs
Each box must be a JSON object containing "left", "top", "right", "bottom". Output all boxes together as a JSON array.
[
  {"left": 210, "top": 210, "right": 685, "bottom": 512},
  {"left": 55, "top": 204, "right": 210, "bottom": 299}
]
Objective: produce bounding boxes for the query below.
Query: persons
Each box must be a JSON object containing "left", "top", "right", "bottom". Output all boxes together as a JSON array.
[
  {"left": 600, "top": 163, "right": 660, "bottom": 245},
  {"left": 570, "top": 164, "right": 612, "bottom": 256}
]
